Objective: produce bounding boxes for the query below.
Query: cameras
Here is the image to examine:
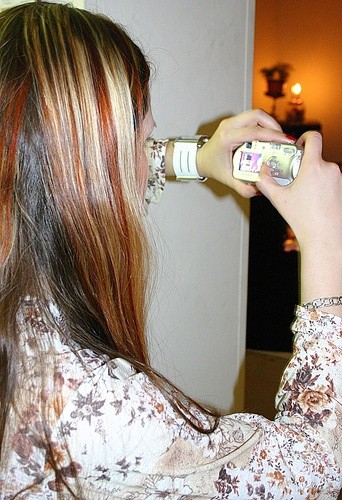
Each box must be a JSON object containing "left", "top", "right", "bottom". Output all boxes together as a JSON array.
[{"left": 232, "top": 140, "right": 304, "bottom": 186}]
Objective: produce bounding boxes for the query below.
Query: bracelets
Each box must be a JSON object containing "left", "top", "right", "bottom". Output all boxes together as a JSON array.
[
  {"left": 173, "top": 135, "right": 211, "bottom": 182},
  {"left": 302, "top": 296, "right": 342, "bottom": 311}
]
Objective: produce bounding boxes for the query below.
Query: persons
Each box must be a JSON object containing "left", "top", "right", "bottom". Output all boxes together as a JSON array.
[{"left": 0, "top": 1, "right": 342, "bottom": 500}]
